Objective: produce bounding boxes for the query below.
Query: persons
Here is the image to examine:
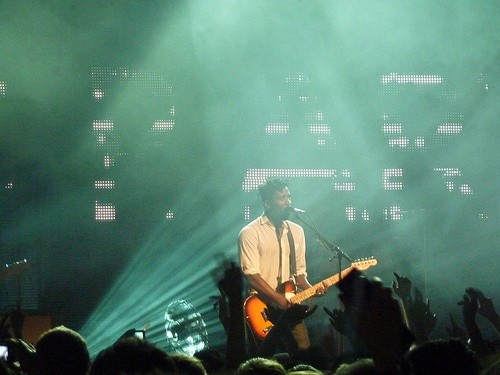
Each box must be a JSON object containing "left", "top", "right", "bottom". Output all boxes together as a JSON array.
[
  {"left": 0, "top": 262, "right": 500, "bottom": 375},
  {"left": 238, "top": 178, "right": 328, "bottom": 361}
]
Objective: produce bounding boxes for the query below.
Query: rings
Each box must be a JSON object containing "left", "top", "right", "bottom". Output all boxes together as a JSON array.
[{"left": 319, "top": 293, "right": 321, "bottom": 295}]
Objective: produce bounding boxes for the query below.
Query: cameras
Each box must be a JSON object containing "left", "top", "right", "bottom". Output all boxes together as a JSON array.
[
  {"left": 135, "top": 330, "right": 145, "bottom": 340},
  {"left": 0, "top": 343, "right": 11, "bottom": 361}
]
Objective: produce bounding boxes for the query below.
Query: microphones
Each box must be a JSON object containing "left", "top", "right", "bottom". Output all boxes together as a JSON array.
[{"left": 289, "top": 207, "right": 305, "bottom": 214}]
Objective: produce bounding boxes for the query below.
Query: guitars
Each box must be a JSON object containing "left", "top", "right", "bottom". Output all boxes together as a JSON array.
[{"left": 243, "top": 256, "right": 377, "bottom": 341}]
